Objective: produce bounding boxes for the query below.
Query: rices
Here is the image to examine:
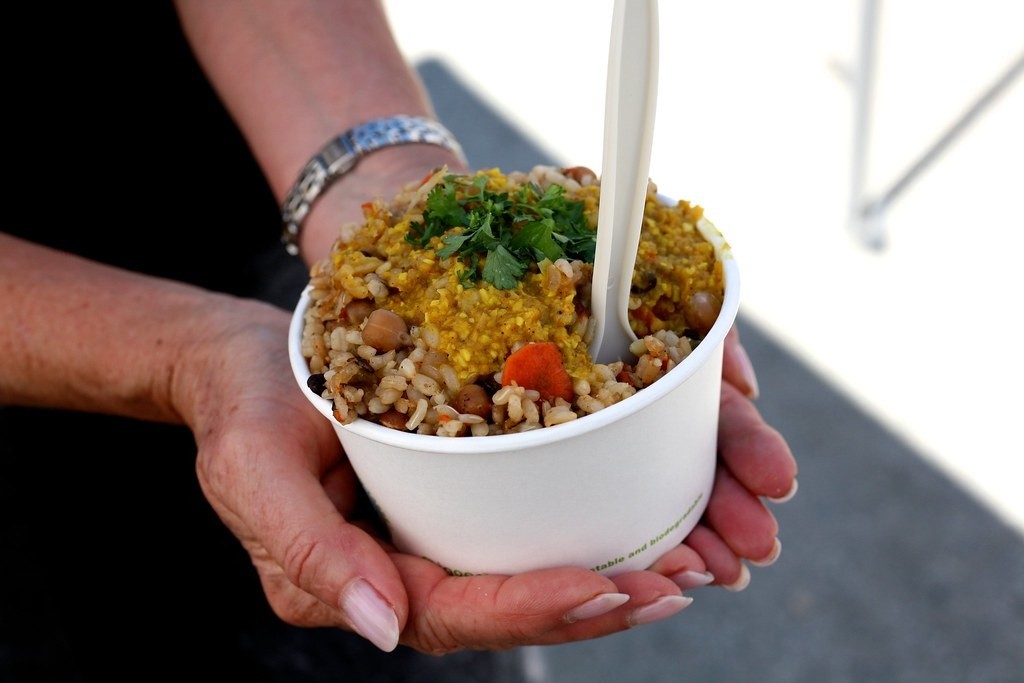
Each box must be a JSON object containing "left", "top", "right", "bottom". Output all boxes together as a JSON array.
[{"left": 296, "top": 167, "right": 722, "bottom": 439}]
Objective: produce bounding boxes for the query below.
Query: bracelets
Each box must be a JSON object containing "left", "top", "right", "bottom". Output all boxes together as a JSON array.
[{"left": 278, "top": 115, "right": 468, "bottom": 255}]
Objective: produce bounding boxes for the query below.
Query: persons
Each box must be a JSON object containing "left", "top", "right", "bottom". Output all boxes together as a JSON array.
[{"left": 1, "top": 0, "right": 801, "bottom": 653}]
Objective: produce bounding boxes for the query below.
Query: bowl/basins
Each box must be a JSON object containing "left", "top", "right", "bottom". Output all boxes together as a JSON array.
[{"left": 286, "top": 187, "right": 744, "bottom": 582}]
